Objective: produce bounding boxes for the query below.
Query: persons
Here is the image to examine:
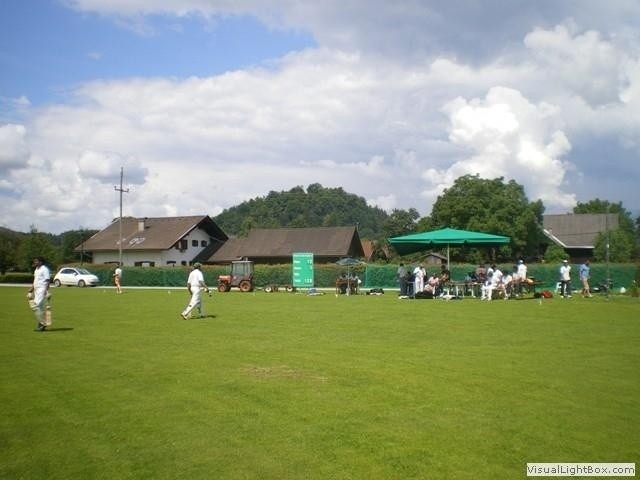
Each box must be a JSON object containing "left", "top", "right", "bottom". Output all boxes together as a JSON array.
[
  {"left": 112, "top": 264, "right": 123, "bottom": 295},
  {"left": 397, "top": 260, "right": 529, "bottom": 302},
  {"left": 349, "top": 271, "right": 359, "bottom": 295},
  {"left": 338, "top": 273, "right": 348, "bottom": 293},
  {"left": 180, "top": 262, "right": 208, "bottom": 320},
  {"left": 579, "top": 260, "right": 594, "bottom": 298},
  {"left": 26, "top": 257, "right": 52, "bottom": 331},
  {"left": 559, "top": 259, "right": 572, "bottom": 299}
]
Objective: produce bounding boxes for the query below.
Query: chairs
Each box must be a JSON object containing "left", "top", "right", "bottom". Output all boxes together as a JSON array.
[{"left": 555, "top": 282, "right": 567, "bottom": 295}]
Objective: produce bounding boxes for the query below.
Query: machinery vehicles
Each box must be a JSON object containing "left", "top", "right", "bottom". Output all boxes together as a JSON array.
[{"left": 216, "top": 261, "right": 295, "bottom": 293}]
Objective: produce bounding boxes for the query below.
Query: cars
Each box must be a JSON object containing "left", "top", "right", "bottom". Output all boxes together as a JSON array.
[{"left": 52, "top": 267, "right": 99, "bottom": 288}]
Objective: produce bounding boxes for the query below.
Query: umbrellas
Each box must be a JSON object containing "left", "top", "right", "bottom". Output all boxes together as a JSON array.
[
  {"left": 336, "top": 256, "right": 364, "bottom": 288},
  {"left": 388, "top": 226, "right": 512, "bottom": 271}
]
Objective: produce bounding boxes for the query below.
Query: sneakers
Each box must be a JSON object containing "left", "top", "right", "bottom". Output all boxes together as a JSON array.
[
  {"left": 181, "top": 312, "right": 206, "bottom": 320},
  {"left": 38, "top": 322, "right": 48, "bottom": 331}
]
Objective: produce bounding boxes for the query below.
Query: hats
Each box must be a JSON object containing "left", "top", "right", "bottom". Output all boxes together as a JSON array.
[{"left": 193, "top": 261, "right": 203, "bottom": 269}]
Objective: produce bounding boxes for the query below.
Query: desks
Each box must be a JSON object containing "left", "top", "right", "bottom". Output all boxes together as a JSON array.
[
  {"left": 407, "top": 281, "right": 478, "bottom": 299},
  {"left": 336, "top": 279, "right": 358, "bottom": 296}
]
